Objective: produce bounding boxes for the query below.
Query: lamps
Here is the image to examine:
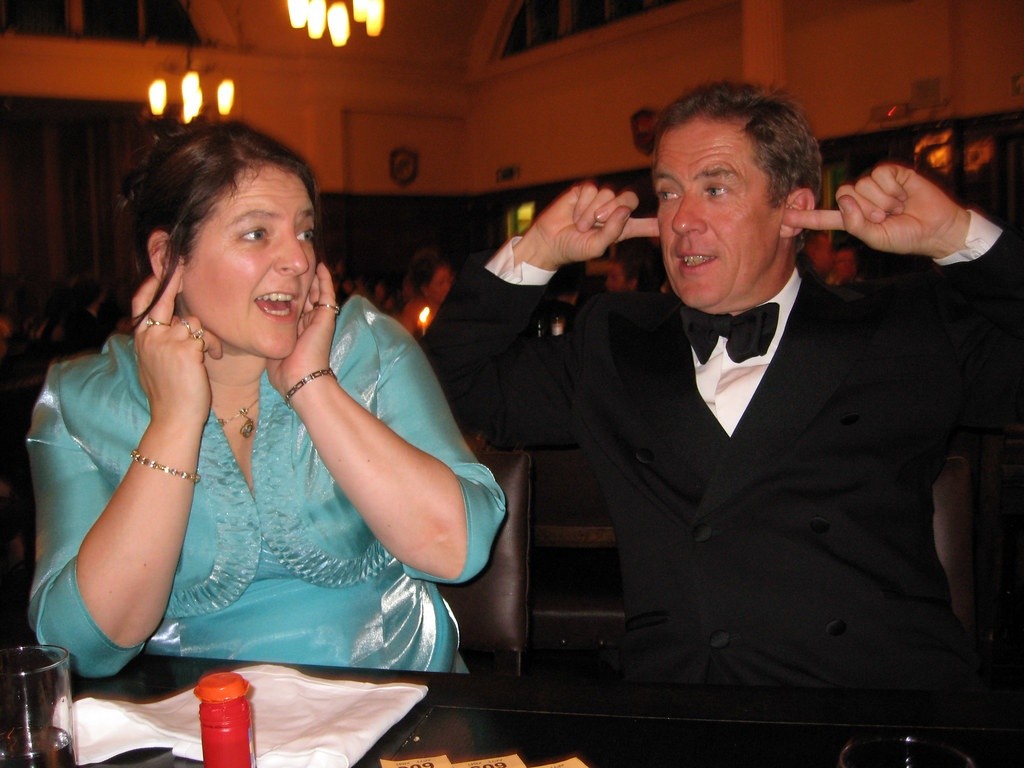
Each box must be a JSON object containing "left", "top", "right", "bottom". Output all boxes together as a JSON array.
[
  {"left": 286, "top": 0, "right": 387, "bottom": 48},
  {"left": 148, "top": 0, "right": 238, "bottom": 128}
]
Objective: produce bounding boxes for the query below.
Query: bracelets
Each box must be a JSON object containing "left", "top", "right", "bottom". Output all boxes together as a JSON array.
[
  {"left": 130, "top": 450, "right": 200, "bottom": 484},
  {"left": 283, "top": 368, "right": 338, "bottom": 409}
]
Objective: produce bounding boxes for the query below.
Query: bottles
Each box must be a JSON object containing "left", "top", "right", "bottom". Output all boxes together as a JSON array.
[{"left": 194, "top": 674, "right": 255, "bottom": 768}]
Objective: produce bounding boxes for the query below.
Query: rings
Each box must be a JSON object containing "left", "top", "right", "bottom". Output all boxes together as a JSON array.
[
  {"left": 191, "top": 328, "right": 205, "bottom": 352},
  {"left": 146, "top": 316, "right": 171, "bottom": 328},
  {"left": 173, "top": 318, "right": 192, "bottom": 338},
  {"left": 310, "top": 302, "right": 341, "bottom": 313}
]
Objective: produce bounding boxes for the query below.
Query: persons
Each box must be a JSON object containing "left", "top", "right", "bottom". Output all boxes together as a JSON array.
[
  {"left": 62, "top": 243, "right": 122, "bottom": 352},
  {"left": 311, "top": 199, "right": 871, "bottom": 333},
  {"left": 25, "top": 119, "right": 506, "bottom": 679},
  {"left": 418, "top": 83, "right": 1024, "bottom": 686}
]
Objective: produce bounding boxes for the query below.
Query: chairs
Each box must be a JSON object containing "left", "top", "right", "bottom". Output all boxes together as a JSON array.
[
  {"left": 931, "top": 455, "right": 978, "bottom": 650},
  {"left": 435, "top": 450, "right": 534, "bottom": 678}
]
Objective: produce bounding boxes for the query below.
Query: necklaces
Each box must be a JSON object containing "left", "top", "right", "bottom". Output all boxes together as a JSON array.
[{"left": 213, "top": 398, "right": 258, "bottom": 438}]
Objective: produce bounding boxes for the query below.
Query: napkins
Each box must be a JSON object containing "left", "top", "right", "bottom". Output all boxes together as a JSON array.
[{"left": 73, "top": 658, "right": 429, "bottom": 768}]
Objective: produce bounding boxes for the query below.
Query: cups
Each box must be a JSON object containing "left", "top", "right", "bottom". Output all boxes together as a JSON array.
[
  {"left": 838, "top": 735, "right": 975, "bottom": 768},
  {"left": 0, "top": 645, "right": 79, "bottom": 768}
]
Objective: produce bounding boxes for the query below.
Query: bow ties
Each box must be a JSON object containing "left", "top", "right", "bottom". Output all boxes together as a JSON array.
[{"left": 681, "top": 303, "right": 781, "bottom": 365}]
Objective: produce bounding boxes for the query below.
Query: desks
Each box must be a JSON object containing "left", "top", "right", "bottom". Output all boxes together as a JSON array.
[{"left": 0, "top": 652, "right": 1024, "bottom": 768}]
